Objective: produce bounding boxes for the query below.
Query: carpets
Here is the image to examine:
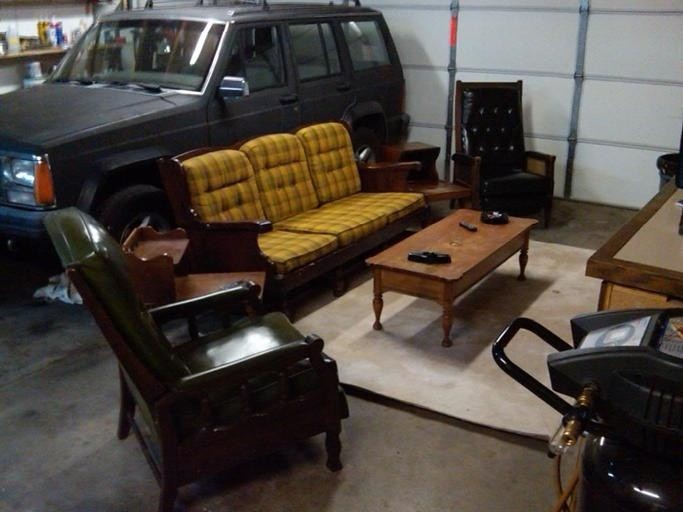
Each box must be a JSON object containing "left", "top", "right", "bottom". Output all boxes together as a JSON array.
[{"left": 292, "top": 237, "right": 603, "bottom": 444}]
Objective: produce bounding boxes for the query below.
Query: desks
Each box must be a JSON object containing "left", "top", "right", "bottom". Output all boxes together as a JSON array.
[{"left": 582, "top": 176, "right": 682, "bottom": 312}]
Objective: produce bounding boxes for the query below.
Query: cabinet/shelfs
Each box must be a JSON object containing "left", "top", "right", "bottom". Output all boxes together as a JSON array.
[
  {"left": 122, "top": 216, "right": 267, "bottom": 307},
  {"left": 383, "top": 142, "right": 472, "bottom": 204}
]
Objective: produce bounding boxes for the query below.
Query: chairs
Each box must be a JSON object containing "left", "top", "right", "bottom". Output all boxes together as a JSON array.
[
  {"left": 452, "top": 78, "right": 555, "bottom": 227},
  {"left": 42, "top": 206, "right": 349, "bottom": 512}
]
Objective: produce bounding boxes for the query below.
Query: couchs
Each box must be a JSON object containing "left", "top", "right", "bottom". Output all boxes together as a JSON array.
[{"left": 157, "top": 117, "right": 434, "bottom": 323}]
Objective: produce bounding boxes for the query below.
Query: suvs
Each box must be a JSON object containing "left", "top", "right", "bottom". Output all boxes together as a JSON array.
[{"left": 0, "top": 0, "right": 409, "bottom": 251}]
